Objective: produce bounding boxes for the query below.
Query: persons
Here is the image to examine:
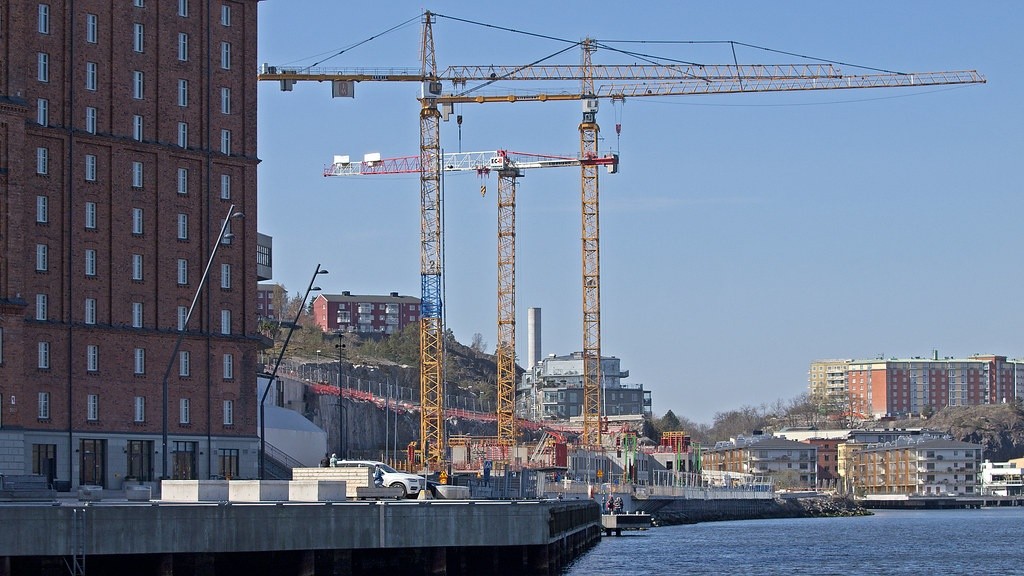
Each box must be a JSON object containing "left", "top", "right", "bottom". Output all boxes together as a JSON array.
[
  {"left": 619, "top": 498, "right": 624, "bottom": 512},
  {"left": 607, "top": 496, "right": 615, "bottom": 513},
  {"left": 373, "top": 464, "right": 385, "bottom": 488},
  {"left": 320, "top": 455, "right": 329, "bottom": 467},
  {"left": 329, "top": 454, "right": 338, "bottom": 467},
  {"left": 615, "top": 498, "right": 621, "bottom": 513}
]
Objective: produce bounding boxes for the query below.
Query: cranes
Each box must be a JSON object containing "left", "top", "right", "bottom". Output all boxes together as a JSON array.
[
  {"left": 426, "top": 33, "right": 986, "bottom": 451},
  {"left": 255, "top": 12, "right": 850, "bottom": 469},
  {"left": 319, "top": 146, "right": 623, "bottom": 466}
]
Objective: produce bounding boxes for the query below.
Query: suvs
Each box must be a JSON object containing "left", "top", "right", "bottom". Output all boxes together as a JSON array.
[{"left": 326, "top": 458, "right": 429, "bottom": 499}]
